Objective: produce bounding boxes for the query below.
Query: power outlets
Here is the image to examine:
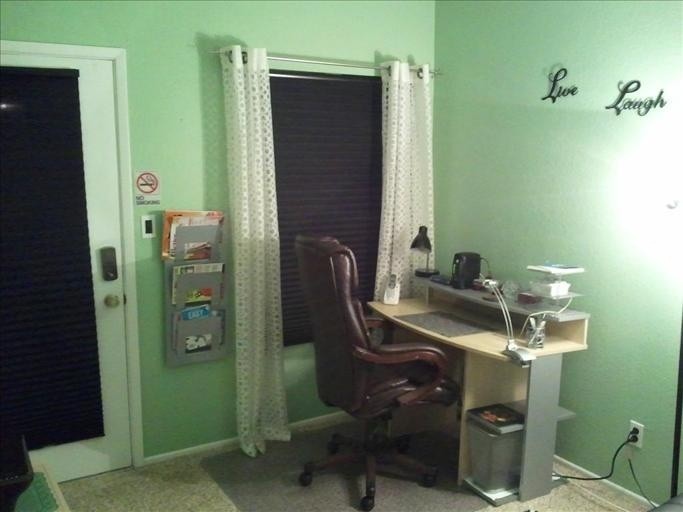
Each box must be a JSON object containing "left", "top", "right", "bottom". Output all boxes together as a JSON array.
[{"left": 627, "top": 419, "right": 645, "bottom": 449}]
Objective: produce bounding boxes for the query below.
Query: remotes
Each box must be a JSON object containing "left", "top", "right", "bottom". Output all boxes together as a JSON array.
[{"left": 431, "top": 276, "right": 450, "bottom": 285}]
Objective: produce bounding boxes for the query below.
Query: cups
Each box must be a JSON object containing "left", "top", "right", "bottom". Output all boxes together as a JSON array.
[{"left": 525, "top": 327, "right": 545, "bottom": 349}]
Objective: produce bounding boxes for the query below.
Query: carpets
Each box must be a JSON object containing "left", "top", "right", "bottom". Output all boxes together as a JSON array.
[
  {"left": 12, "top": 458, "right": 72, "bottom": 511},
  {"left": 199, "top": 422, "right": 570, "bottom": 510}
]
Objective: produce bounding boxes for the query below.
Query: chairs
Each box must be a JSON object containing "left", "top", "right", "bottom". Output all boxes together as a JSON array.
[{"left": 294, "top": 233, "right": 461, "bottom": 511}]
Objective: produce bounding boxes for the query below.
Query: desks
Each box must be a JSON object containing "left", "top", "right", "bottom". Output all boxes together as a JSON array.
[{"left": 365, "top": 264, "right": 592, "bottom": 507}]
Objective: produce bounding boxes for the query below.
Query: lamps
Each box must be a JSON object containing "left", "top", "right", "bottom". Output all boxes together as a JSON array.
[{"left": 407, "top": 225, "right": 440, "bottom": 279}]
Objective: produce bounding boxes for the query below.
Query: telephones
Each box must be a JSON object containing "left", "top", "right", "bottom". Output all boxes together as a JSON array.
[{"left": 383, "top": 274, "right": 400, "bottom": 306}]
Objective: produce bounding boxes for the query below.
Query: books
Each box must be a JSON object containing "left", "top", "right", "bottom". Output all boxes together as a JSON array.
[
  {"left": 524, "top": 265, "right": 586, "bottom": 275},
  {"left": 465, "top": 401, "right": 526, "bottom": 432},
  {"left": 158, "top": 206, "right": 227, "bottom": 356}
]
